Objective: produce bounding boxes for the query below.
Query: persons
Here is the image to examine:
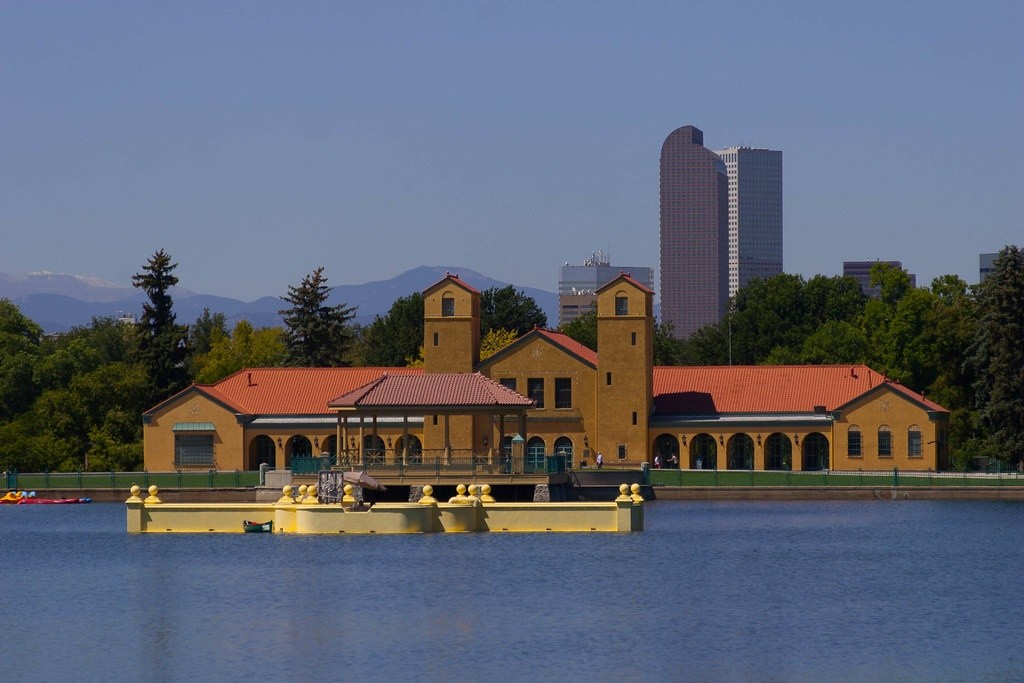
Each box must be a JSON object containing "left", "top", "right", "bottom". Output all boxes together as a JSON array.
[
  {"left": 597, "top": 452, "right": 602, "bottom": 468},
  {"left": 667, "top": 453, "right": 677, "bottom": 468},
  {"left": 696, "top": 453, "right": 703, "bottom": 469},
  {"left": 655, "top": 456, "right": 660, "bottom": 470}
]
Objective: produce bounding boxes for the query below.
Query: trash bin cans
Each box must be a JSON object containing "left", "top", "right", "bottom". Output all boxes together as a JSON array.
[{"left": 696, "top": 458, "right": 704, "bottom": 469}]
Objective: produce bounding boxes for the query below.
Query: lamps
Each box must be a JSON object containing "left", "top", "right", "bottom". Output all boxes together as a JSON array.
[
  {"left": 719, "top": 434, "right": 724, "bottom": 444},
  {"left": 682, "top": 435, "right": 686, "bottom": 445},
  {"left": 484, "top": 436, "right": 487, "bottom": 446},
  {"left": 277, "top": 438, "right": 281, "bottom": 447},
  {"left": 584, "top": 435, "right": 588, "bottom": 445},
  {"left": 314, "top": 437, "right": 318, "bottom": 446},
  {"left": 757, "top": 434, "right": 761, "bottom": 444},
  {"left": 387, "top": 436, "right": 391, "bottom": 446},
  {"left": 350, "top": 435, "right": 355, "bottom": 445},
  {"left": 794, "top": 434, "right": 799, "bottom": 442}
]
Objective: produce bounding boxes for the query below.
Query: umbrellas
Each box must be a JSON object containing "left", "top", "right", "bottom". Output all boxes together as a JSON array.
[{"left": 343, "top": 470, "right": 388, "bottom": 507}]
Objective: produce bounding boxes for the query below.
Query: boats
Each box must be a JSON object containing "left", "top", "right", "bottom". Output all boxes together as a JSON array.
[
  {"left": 0, "top": 489, "right": 92, "bottom": 505},
  {"left": 243, "top": 519, "right": 273, "bottom": 534}
]
